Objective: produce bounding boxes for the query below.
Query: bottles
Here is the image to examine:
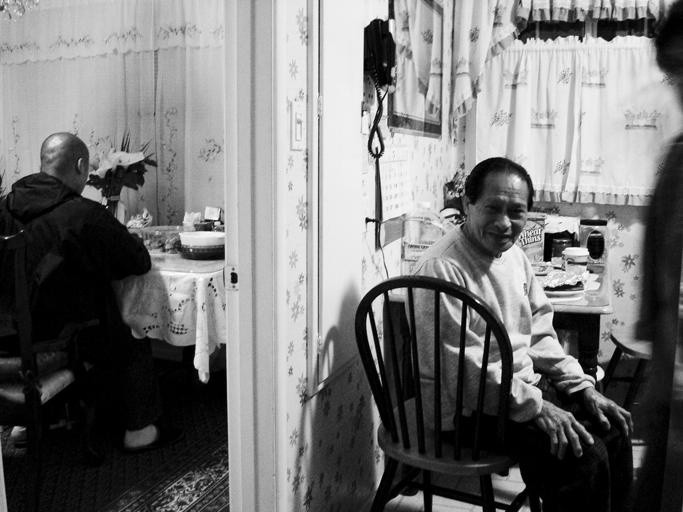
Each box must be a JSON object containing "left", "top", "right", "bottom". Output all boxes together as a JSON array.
[
  {"left": 141, "top": 225, "right": 177, "bottom": 256},
  {"left": 552, "top": 236, "right": 570, "bottom": 256}
]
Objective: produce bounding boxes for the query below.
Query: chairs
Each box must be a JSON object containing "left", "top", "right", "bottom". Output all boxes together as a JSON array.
[
  {"left": 350, "top": 274, "right": 540, "bottom": 511},
  {"left": 0, "top": 228, "right": 102, "bottom": 512}
]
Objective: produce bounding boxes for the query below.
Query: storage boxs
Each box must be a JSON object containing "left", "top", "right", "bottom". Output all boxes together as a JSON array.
[{"left": 513, "top": 212, "right": 549, "bottom": 265}]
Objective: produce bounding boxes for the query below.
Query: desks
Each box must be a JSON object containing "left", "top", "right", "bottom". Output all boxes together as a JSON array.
[
  {"left": 91, "top": 216, "right": 224, "bottom": 387},
  {"left": 387, "top": 235, "right": 614, "bottom": 481}
]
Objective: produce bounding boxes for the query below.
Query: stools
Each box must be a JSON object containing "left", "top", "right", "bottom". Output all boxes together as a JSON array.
[{"left": 602, "top": 330, "right": 654, "bottom": 412}]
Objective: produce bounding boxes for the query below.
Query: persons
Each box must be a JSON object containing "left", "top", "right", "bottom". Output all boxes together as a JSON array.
[
  {"left": 624, "top": 7, "right": 681, "bottom": 511},
  {"left": 0, "top": 132, "right": 185, "bottom": 454},
  {"left": 403, "top": 157, "right": 636, "bottom": 509}
]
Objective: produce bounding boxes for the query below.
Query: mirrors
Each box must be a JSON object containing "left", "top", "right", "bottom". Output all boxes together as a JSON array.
[{"left": 386, "top": 0, "right": 446, "bottom": 139}]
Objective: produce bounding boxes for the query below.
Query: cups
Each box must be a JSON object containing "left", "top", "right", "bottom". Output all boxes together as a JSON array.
[{"left": 563, "top": 246, "right": 588, "bottom": 271}]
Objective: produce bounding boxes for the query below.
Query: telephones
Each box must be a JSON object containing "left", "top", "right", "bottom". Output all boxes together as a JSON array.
[{"left": 364, "top": 18, "right": 396, "bottom": 87}]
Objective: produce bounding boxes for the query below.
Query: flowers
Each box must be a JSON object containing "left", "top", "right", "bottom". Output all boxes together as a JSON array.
[{"left": 85, "top": 126, "right": 160, "bottom": 215}]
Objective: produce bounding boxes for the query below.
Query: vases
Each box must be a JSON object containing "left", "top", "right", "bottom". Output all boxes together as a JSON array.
[{"left": 97, "top": 195, "right": 130, "bottom": 228}]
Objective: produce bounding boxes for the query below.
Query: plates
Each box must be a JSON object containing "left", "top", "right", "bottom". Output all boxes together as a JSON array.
[{"left": 532, "top": 265, "right": 595, "bottom": 295}]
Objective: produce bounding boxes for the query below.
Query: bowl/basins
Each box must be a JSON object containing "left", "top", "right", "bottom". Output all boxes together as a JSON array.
[{"left": 178, "top": 230, "right": 224, "bottom": 260}]
